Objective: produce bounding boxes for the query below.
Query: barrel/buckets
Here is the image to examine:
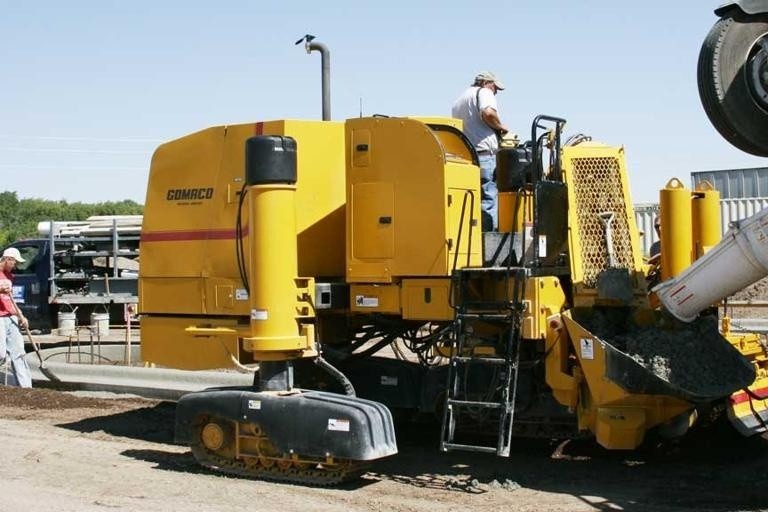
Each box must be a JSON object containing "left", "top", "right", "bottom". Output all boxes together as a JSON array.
[
  {"left": 58, "top": 312, "right": 76, "bottom": 337},
  {"left": 90, "top": 311, "right": 110, "bottom": 337}
]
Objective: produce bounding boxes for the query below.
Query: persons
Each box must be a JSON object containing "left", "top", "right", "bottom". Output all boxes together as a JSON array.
[
  {"left": 450, "top": 70, "right": 509, "bottom": 229},
  {"left": 1, "top": 246, "right": 34, "bottom": 389},
  {"left": 648, "top": 214, "right": 662, "bottom": 264}
]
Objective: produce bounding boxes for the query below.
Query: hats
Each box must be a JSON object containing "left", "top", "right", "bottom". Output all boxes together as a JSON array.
[{"left": 4, "top": 248, "right": 27, "bottom": 264}]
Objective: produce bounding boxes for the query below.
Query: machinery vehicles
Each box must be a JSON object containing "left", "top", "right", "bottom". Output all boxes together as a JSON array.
[{"left": 136, "top": 0, "right": 767, "bottom": 490}]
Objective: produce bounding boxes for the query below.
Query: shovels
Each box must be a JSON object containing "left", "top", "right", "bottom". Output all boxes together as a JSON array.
[{"left": 4, "top": 289, "right": 61, "bottom": 383}]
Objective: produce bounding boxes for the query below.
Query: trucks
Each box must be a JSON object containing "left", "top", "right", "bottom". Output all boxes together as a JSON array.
[{"left": 0, "top": 232, "right": 140, "bottom": 332}]
